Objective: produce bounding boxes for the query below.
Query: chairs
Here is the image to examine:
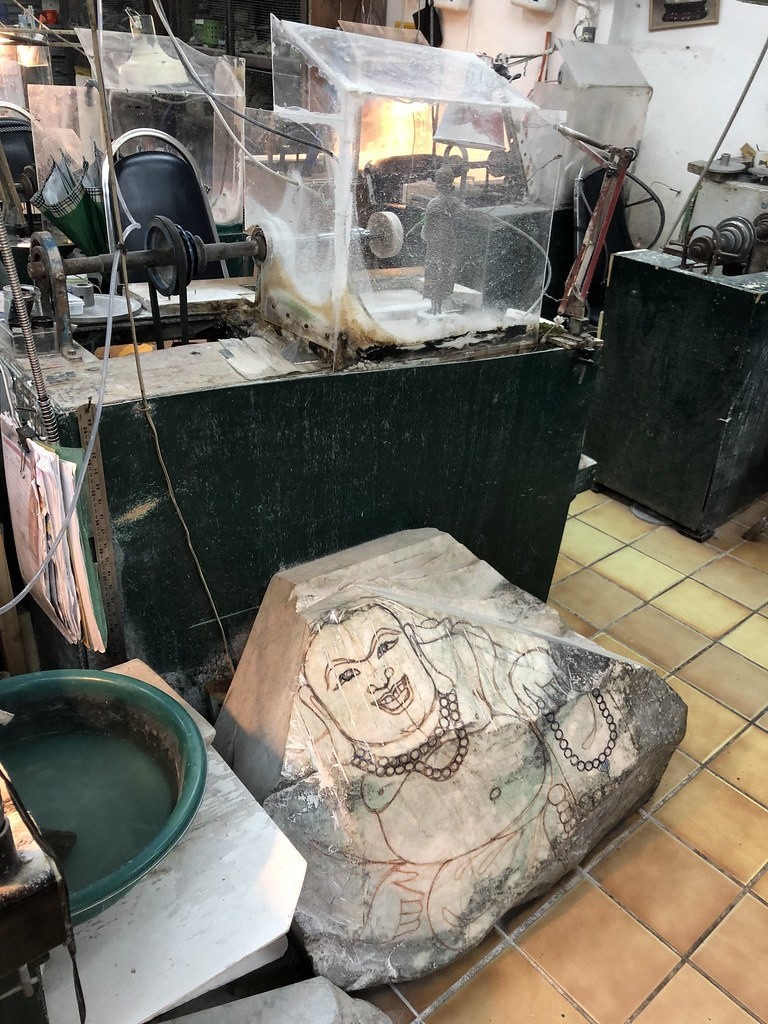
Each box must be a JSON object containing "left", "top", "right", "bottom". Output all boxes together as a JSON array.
[
  {"left": 578, "top": 149, "right": 645, "bottom": 310},
  {"left": 0, "top": 99, "right": 71, "bottom": 221},
  {"left": 103, "top": 122, "right": 236, "bottom": 301}
]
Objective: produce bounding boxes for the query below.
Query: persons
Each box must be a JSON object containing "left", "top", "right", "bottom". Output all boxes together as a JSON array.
[{"left": 420, "top": 168, "right": 474, "bottom": 315}]
